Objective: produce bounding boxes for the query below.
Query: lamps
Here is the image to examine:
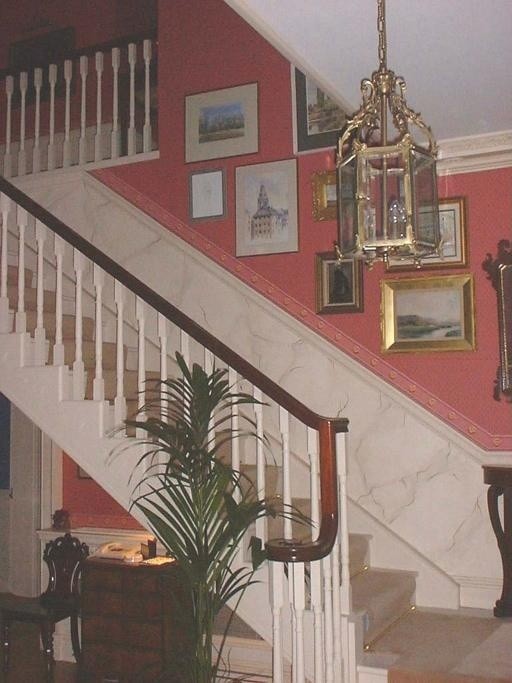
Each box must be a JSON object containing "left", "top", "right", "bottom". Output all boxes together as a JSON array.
[{"left": 331, "top": 0, "right": 446, "bottom": 270}]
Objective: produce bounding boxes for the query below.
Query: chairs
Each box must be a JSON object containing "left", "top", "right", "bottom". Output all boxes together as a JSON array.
[{"left": 0, "top": 533, "right": 89, "bottom": 683}]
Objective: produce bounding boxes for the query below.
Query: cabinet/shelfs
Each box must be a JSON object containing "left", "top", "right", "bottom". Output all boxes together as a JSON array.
[{"left": 81, "top": 551, "right": 196, "bottom": 683}]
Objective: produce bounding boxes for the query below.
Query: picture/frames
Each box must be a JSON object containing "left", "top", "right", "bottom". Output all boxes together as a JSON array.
[
  {"left": 290, "top": 61, "right": 354, "bottom": 156},
  {"left": 188, "top": 166, "right": 228, "bottom": 222},
  {"left": 315, "top": 252, "right": 365, "bottom": 315},
  {"left": 233, "top": 157, "right": 300, "bottom": 259},
  {"left": 377, "top": 274, "right": 477, "bottom": 356},
  {"left": 386, "top": 197, "right": 470, "bottom": 272},
  {"left": 183, "top": 81, "right": 260, "bottom": 164},
  {"left": 312, "top": 170, "right": 360, "bottom": 222}
]
